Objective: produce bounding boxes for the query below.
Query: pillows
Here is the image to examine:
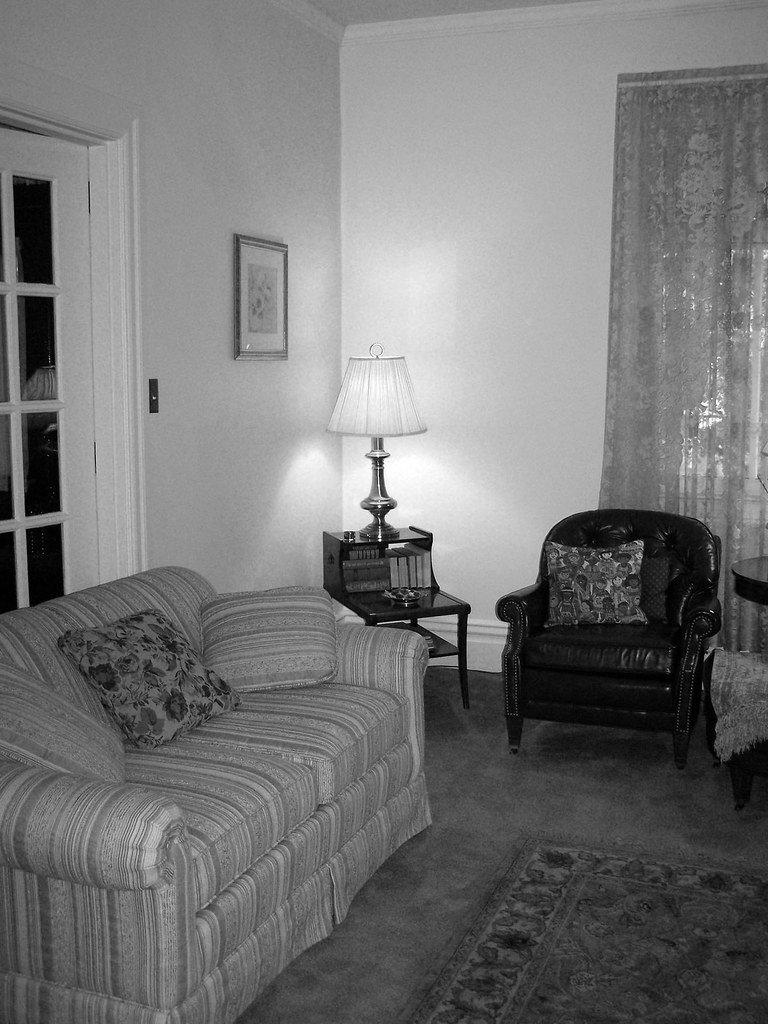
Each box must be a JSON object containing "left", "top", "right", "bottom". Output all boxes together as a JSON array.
[
  {"left": 197, "top": 585, "right": 339, "bottom": 693},
  {"left": 57, "top": 608, "right": 243, "bottom": 750},
  {"left": 0, "top": 657, "right": 126, "bottom": 784},
  {"left": 542, "top": 539, "right": 648, "bottom": 629},
  {"left": 640, "top": 556, "right": 671, "bottom": 625}
]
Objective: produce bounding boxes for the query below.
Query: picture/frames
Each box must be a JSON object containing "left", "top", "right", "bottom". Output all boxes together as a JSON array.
[{"left": 233, "top": 232, "right": 289, "bottom": 362}]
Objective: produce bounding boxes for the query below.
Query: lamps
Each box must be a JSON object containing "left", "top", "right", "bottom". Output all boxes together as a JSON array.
[
  {"left": 324, "top": 342, "right": 428, "bottom": 539},
  {"left": 22, "top": 358, "right": 58, "bottom": 400}
]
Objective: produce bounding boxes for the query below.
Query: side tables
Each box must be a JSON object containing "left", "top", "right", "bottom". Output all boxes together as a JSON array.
[{"left": 322, "top": 525, "right": 471, "bottom": 710}]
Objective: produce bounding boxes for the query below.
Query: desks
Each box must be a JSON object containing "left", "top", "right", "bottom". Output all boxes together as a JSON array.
[{"left": 730, "top": 556, "right": 768, "bottom": 605}]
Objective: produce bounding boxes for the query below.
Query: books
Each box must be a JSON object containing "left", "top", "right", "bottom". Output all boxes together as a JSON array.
[{"left": 383, "top": 543, "right": 431, "bottom": 589}]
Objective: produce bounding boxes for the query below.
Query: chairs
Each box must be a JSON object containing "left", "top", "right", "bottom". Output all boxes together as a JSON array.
[{"left": 495, "top": 508, "right": 722, "bottom": 770}]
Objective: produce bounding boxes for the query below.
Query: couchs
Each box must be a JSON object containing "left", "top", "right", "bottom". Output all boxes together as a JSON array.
[{"left": 0, "top": 565, "right": 432, "bottom": 1024}]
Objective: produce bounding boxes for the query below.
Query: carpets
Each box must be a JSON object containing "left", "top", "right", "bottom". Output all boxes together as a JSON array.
[{"left": 394, "top": 830, "right": 768, "bottom": 1024}]
